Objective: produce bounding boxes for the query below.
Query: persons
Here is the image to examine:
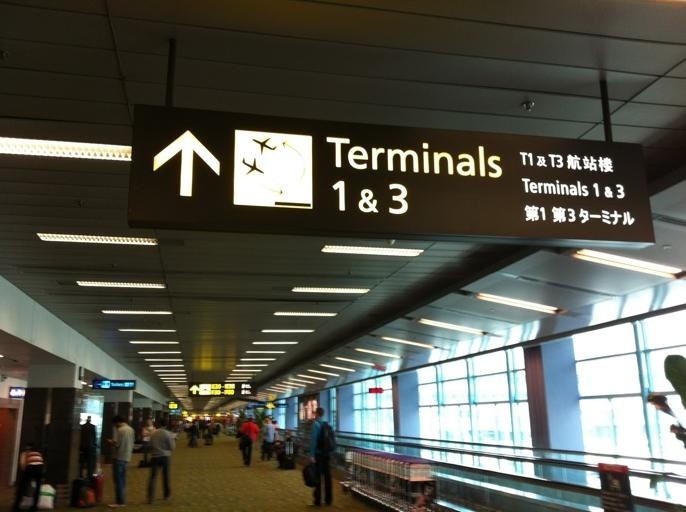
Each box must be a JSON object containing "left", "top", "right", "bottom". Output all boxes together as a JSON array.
[
  {"left": 305, "top": 406, "right": 334, "bottom": 509},
  {"left": 179, "top": 415, "right": 299, "bottom": 462},
  {"left": 10, "top": 410, "right": 180, "bottom": 512},
  {"left": 238, "top": 417, "right": 260, "bottom": 466}
]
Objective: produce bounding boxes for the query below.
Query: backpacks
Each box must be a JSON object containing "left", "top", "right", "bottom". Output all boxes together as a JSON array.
[{"left": 316, "top": 419, "right": 336, "bottom": 452}]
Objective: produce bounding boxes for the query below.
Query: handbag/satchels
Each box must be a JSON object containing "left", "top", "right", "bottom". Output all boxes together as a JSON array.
[
  {"left": 239, "top": 434, "right": 252, "bottom": 450},
  {"left": 72, "top": 473, "right": 104, "bottom": 508},
  {"left": 305, "top": 463, "right": 320, "bottom": 487},
  {"left": 280, "top": 455, "right": 295, "bottom": 468},
  {"left": 37, "top": 484, "right": 56, "bottom": 510}
]
